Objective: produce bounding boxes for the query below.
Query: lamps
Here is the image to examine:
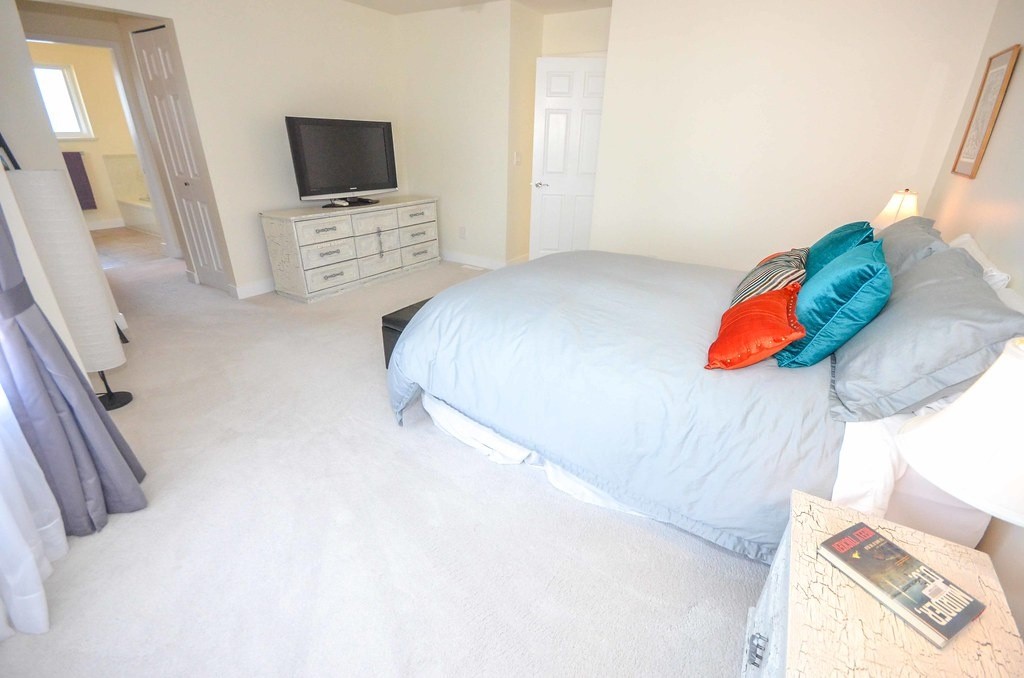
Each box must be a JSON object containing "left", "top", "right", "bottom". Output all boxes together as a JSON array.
[
  {"left": 895, "top": 337, "right": 1024, "bottom": 529},
  {"left": 877, "top": 188, "right": 918, "bottom": 224},
  {"left": 8, "top": 170, "right": 134, "bottom": 411}
]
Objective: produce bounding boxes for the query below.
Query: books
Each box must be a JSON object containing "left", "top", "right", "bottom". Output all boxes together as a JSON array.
[{"left": 817, "top": 522, "right": 986, "bottom": 651}]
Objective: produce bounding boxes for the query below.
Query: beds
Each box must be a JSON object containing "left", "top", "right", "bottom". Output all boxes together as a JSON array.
[{"left": 387, "top": 232, "right": 1024, "bottom": 569}]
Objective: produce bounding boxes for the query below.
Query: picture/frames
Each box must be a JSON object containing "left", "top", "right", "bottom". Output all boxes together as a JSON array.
[{"left": 952, "top": 43, "right": 1021, "bottom": 179}]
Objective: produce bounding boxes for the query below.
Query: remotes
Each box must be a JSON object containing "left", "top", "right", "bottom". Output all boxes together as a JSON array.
[{"left": 331, "top": 198, "right": 349, "bottom": 206}]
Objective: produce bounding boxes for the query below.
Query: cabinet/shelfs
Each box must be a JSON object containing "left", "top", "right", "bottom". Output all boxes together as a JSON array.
[{"left": 258, "top": 196, "right": 442, "bottom": 305}]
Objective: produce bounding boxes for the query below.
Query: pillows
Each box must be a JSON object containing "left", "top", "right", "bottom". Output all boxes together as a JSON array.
[
  {"left": 704, "top": 282, "right": 807, "bottom": 369},
  {"left": 800, "top": 221, "right": 874, "bottom": 288},
  {"left": 773, "top": 237, "right": 892, "bottom": 367},
  {"left": 757, "top": 247, "right": 794, "bottom": 266},
  {"left": 829, "top": 248, "right": 1024, "bottom": 421},
  {"left": 874, "top": 217, "right": 951, "bottom": 277},
  {"left": 730, "top": 249, "right": 809, "bottom": 309}
]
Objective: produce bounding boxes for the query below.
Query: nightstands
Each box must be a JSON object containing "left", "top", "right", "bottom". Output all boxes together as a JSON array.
[{"left": 741, "top": 491, "right": 1024, "bottom": 678}]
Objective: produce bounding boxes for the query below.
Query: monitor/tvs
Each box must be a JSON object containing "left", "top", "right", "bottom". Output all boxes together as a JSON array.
[{"left": 285, "top": 116, "right": 399, "bottom": 209}]
